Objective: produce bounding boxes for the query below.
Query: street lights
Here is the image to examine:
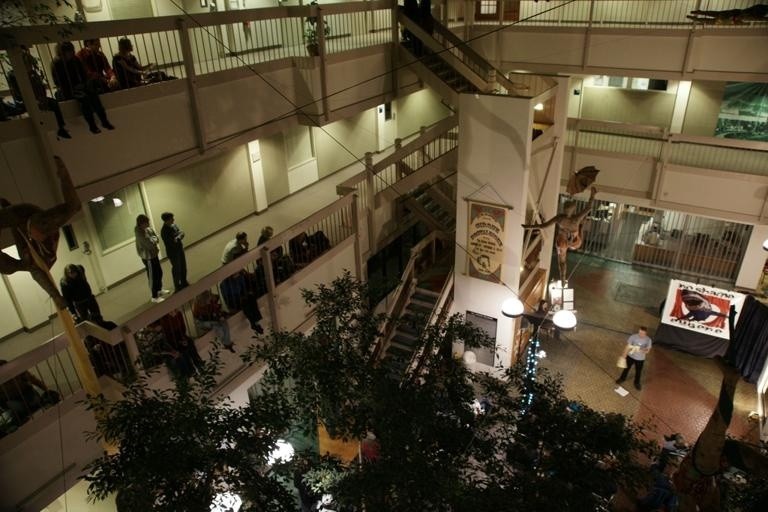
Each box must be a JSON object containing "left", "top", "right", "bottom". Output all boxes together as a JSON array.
[
  {"left": 500, "top": 278, "right": 578, "bottom": 420},
  {"left": 208, "top": 434, "right": 349, "bottom": 511}
]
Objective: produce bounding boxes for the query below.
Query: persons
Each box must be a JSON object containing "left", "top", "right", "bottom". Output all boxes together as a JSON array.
[
  {"left": 134, "top": 214, "right": 171, "bottom": 302},
  {"left": 0, "top": 360, "right": 49, "bottom": 438},
  {"left": 143, "top": 291, "right": 236, "bottom": 384},
  {"left": 615, "top": 325, "right": 653, "bottom": 391},
  {"left": 521, "top": 186, "right": 600, "bottom": 285},
  {"left": 218, "top": 226, "right": 330, "bottom": 335},
  {"left": 0, "top": 34, "right": 178, "bottom": 142},
  {"left": 160, "top": 213, "right": 189, "bottom": 292},
  {"left": 60, "top": 263, "right": 136, "bottom": 383}
]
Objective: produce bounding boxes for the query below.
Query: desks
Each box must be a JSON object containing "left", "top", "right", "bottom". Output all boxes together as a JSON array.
[{"left": 632, "top": 223, "right": 738, "bottom": 280}]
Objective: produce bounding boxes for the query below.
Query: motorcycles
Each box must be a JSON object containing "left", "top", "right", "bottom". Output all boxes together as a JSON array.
[{"left": 624, "top": 445, "right": 686, "bottom": 511}]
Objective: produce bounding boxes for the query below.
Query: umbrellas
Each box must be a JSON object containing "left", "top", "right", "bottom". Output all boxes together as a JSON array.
[{"left": 564, "top": 165, "right": 599, "bottom": 193}]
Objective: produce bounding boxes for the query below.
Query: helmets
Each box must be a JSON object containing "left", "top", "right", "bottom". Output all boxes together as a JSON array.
[
  {"left": 65, "top": 264, "right": 78, "bottom": 280},
  {"left": 261, "top": 226, "right": 272, "bottom": 238}
]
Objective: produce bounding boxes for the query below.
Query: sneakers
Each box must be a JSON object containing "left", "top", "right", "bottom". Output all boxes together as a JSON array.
[
  {"left": 57, "top": 123, "right": 113, "bottom": 138},
  {"left": 615, "top": 378, "right": 622, "bottom": 384},
  {"left": 152, "top": 297, "right": 164, "bottom": 303},
  {"left": 634, "top": 384, "right": 640, "bottom": 389},
  {"left": 157, "top": 289, "right": 169, "bottom": 295}
]
